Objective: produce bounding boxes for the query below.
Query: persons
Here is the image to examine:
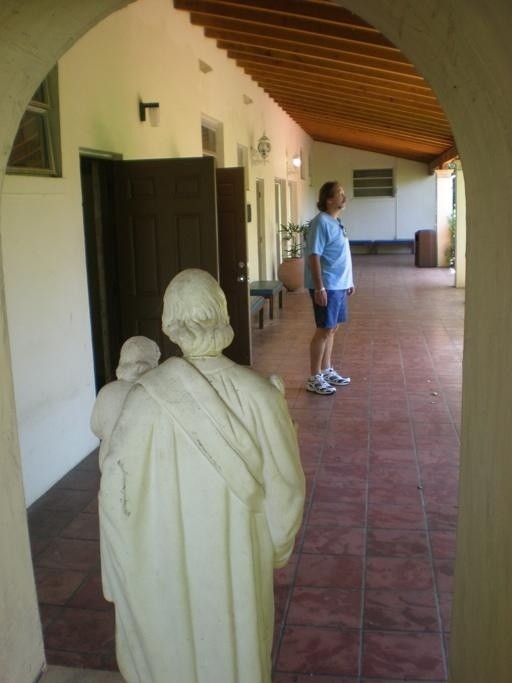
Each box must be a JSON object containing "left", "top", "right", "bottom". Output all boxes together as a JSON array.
[
  {"left": 90, "top": 268, "right": 306, "bottom": 683},
  {"left": 304, "top": 181, "right": 355, "bottom": 395}
]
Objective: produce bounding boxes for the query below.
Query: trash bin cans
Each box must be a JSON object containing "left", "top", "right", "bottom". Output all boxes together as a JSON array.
[{"left": 414, "top": 230, "right": 437, "bottom": 267}]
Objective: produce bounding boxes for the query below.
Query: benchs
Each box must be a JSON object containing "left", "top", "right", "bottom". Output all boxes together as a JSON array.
[{"left": 250, "top": 281, "right": 283, "bottom": 329}]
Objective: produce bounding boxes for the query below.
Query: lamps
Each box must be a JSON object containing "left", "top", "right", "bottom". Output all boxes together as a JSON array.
[
  {"left": 140, "top": 103, "right": 160, "bottom": 127},
  {"left": 250, "top": 136, "right": 272, "bottom": 164}
]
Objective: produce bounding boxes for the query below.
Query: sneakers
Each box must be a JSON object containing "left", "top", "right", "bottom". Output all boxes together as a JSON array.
[
  {"left": 305, "top": 374, "right": 336, "bottom": 395},
  {"left": 322, "top": 369, "right": 351, "bottom": 387}
]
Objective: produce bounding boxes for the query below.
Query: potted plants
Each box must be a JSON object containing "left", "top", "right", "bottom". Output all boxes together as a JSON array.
[{"left": 277, "top": 223, "right": 307, "bottom": 294}]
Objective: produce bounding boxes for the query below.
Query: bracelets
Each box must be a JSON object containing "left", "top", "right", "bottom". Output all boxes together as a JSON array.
[{"left": 314, "top": 288, "right": 325, "bottom": 293}]
